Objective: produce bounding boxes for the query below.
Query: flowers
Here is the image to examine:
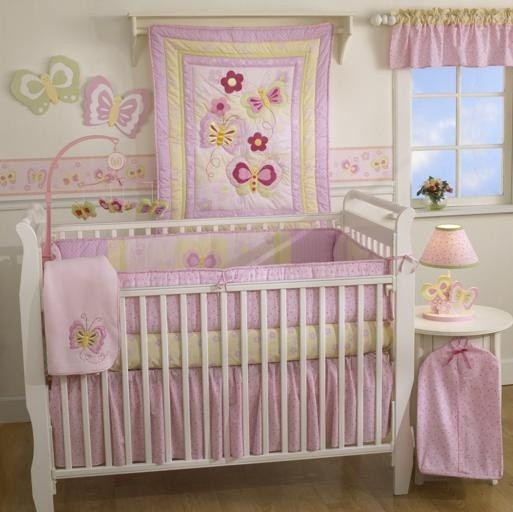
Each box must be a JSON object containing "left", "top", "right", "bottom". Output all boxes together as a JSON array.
[{"left": 416, "top": 175, "right": 454, "bottom": 197}]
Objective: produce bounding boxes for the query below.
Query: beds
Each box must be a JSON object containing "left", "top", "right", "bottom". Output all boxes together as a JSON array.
[{"left": 13, "top": 187, "right": 419, "bottom": 512}]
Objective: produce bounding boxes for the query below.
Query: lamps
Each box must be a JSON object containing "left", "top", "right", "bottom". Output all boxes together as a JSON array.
[{"left": 417, "top": 221, "right": 482, "bottom": 322}]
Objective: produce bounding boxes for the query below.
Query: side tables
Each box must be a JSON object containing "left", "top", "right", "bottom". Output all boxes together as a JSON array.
[{"left": 415, "top": 305, "right": 512, "bottom": 488}]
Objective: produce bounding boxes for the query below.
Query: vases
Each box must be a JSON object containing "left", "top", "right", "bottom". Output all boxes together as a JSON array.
[{"left": 424, "top": 210, "right": 447, "bottom": 211}]
